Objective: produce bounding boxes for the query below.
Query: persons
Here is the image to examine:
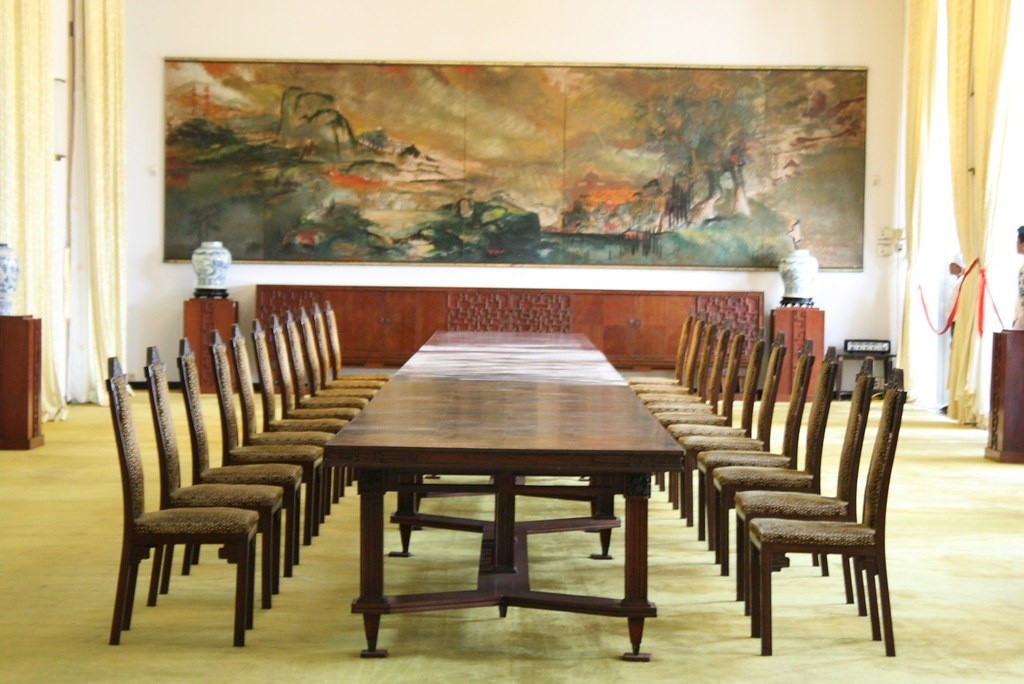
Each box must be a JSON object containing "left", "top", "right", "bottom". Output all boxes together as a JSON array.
[
  {"left": 1014, "top": 227, "right": 1024, "bottom": 333},
  {"left": 938, "top": 252, "right": 965, "bottom": 414}
]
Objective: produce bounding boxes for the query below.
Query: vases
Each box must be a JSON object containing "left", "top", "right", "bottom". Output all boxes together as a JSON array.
[
  {"left": 1014, "top": 261, "right": 1024, "bottom": 330},
  {"left": 778, "top": 249, "right": 818, "bottom": 300},
  {"left": 0, "top": 244, "right": 19, "bottom": 315},
  {"left": 192, "top": 240, "right": 232, "bottom": 288}
]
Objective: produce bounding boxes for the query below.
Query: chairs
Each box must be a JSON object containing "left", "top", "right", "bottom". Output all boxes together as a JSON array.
[
  {"left": 710, "top": 346, "right": 839, "bottom": 578},
  {"left": 176, "top": 337, "right": 304, "bottom": 580},
  {"left": 735, "top": 355, "right": 877, "bottom": 616},
  {"left": 211, "top": 329, "right": 323, "bottom": 547},
  {"left": 696, "top": 338, "right": 817, "bottom": 553},
  {"left": 252, "top": 301, "right": 395, "bottom": 500},
  {"left": 623, "top": 316, "right": 751, "bottom": 515},
  {"left": 230, "top": 323, "right": 336, "bottom": 524},
  {"left": 748, "top": 367, "right": 906, "bottom": 656},
  {"left": 107, "top": 356, "right": 259, "bottom": 648},
  {"left": 145, "top": 346, "right": 285, "bottom": 610},
  {"left": 680, "top": 333, "right": 786, "bottom": 526}
]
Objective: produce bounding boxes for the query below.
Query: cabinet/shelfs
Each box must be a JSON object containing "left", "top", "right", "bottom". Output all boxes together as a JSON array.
[
  {"left": 770, "top": 309, "right": 825, "bottom": 401},
  {"left": 257, "top": 283, "right": 765, "bottom": 374},
  {"left": 184, "top": 297, "right": 239, "bottom": 393},
  {"left": 984, "top": 330, "right": 1024, "bottom": 464},
  {"left": 0, "top": 314, "right": 43, "bottom": 450}
]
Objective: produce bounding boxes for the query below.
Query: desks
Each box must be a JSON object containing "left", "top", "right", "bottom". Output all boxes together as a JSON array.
[{"left": 324, "top": 329, "right": 687, "bottom": 661}]
